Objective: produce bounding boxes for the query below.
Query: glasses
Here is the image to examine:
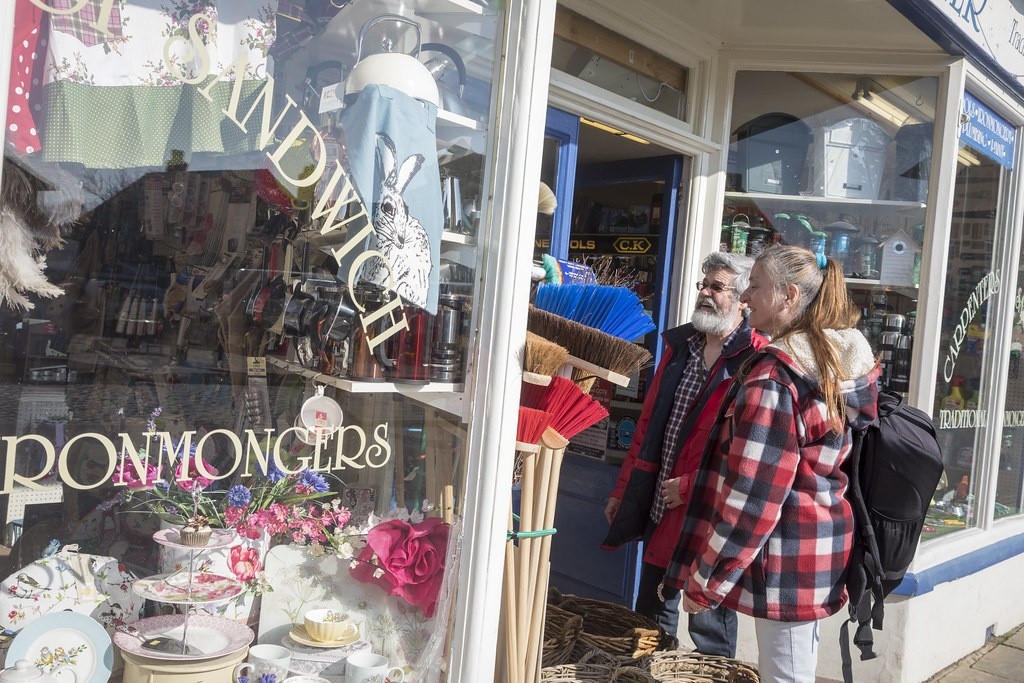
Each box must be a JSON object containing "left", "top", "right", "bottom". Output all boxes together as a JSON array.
[{"left": 696, "top": 279, "right": 739, "bottom": 292}]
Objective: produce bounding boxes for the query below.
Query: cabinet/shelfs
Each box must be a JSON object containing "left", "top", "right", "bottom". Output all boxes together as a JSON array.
[{"left": 719, "top": 191, "right": 925, "bottom": 294}]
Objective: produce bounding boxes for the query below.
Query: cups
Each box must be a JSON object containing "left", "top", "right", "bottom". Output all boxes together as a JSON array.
[
  {"left": 293, "top": 384, "right": 344, "bottom": 446},
  {"left": 344, "top": 652, "right": 405, "bottom": 683},
  {"left": 282, "top": 675, "right": 331, "bottom": 683},
  {"left": 233, "top": 644, "right": 291, "bottom": 683},
  {"left": 304, "top": 608, "right": 358, "bottom": 641}
]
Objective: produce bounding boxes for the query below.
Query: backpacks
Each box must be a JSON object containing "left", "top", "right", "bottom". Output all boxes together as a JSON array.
[{"left": 841, "top": 390, "right": 945, "bottom": 683}]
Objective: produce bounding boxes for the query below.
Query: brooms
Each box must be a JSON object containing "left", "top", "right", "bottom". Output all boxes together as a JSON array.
[{"left": 403, "top": 276, "right": 660, "bottom": 683}]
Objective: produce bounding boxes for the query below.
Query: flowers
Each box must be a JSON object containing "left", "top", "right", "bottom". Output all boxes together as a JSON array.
[{"left": 95, "top": 407, "right": 451, "bottom": 622}]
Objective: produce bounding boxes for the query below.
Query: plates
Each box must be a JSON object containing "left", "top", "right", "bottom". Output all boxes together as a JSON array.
[
  {"left": 289, "top": 624, "right": 361, "bottom": 648},
  {"left": 4, "top": 611, "right": 114, "bottom": 683},
  {"left": 282, "top": 635, "right": 372, "bottom": 660}
]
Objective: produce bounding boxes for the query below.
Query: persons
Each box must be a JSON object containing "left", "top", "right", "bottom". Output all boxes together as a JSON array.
[
  {"left": 657, "top": 246, "right": 850, "bottom": 683},
  {"left": 604, "top": 252, "right": 770, "bottom": 660}
]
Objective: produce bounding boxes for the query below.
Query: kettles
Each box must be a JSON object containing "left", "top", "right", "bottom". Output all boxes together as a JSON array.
[
  {"left": 304, "top": 12, "right": 471, "bottom": 119},
  {"left": 348, "top": 285, "right": 465, "bottom": 385}
]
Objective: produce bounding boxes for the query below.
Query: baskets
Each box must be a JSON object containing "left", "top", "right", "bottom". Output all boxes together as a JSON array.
[{"left": 542, "top": 589, "right": 763, "bottom": 683}]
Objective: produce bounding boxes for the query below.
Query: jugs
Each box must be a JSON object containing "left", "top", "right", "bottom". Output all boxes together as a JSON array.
[{"left": 0, "top": 659, "right": 79, "bottom": 683}]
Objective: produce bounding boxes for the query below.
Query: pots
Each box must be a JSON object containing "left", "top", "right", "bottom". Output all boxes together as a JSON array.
[
  {"left": 245, "top": 276, "right": 358, "bottom": 355},
  {"left": 856, "top": 294, "right": 916, "bottom": 393}
]
